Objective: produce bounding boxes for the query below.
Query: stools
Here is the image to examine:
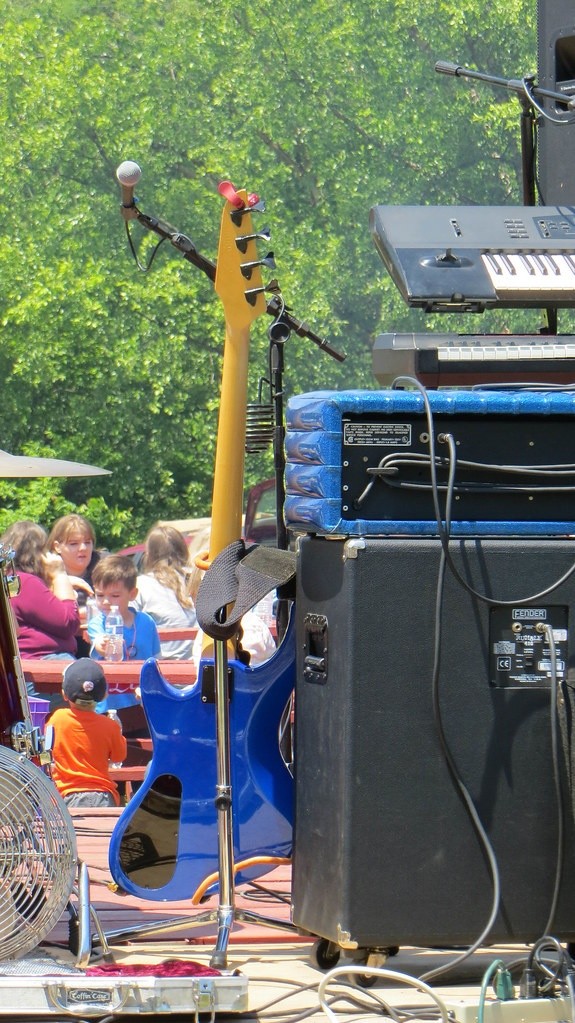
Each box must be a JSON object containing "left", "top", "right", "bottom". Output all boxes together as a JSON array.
[{"left": 83, "top": 737, "right": 153, "bottom": 804}]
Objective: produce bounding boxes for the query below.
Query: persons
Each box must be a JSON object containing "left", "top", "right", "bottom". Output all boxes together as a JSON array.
[
  {"left": 0, "top": 513, "right": 278, "bottom": 818},
  {"left": 43, "top": 656, "right": 127, "bottom": 808}
]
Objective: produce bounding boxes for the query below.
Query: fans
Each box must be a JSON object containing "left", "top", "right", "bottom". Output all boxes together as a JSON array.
[{"left": 0, "top": 746, "right": 77, "bottom": 957}]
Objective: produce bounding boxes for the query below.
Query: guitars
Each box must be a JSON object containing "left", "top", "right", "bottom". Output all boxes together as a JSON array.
[{"left": 109, "top": 178, "right": 297, "bottom": 905}]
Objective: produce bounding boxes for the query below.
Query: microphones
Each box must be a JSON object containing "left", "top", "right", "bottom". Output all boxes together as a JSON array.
[{"left": 115, "top": 160, "right": 142, "bottom": 221}]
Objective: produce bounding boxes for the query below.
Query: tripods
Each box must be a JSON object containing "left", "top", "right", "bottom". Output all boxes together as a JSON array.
[{"left": 91, "top": 198, "right": 346, "bottom": 970}]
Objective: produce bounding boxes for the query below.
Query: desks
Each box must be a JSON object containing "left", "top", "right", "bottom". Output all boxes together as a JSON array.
[{"left": 20, "top": 624, "right": 295, "bottom": 791}]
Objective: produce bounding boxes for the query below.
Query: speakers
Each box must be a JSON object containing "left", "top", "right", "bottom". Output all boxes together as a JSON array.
[
  {"left": 535, "top": 0, "right": 575, "bottom": 208},
  {"left": 293, "top": 530, "right": 575, "bottom": 950}
]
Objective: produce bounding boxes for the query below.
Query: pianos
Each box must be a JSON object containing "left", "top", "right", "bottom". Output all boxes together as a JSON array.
[
  {"left": 373, "top": 332, "right": 575, "bottom": 390},
  {"left": 369, "top": 205, "right": 575, "bottom": 313}
]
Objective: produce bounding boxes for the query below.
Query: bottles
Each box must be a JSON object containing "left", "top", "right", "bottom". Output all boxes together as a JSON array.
[
  {"left": 104, "top": 605, "right": 124, "bottom": 662},
  {"left": 107, "top": 709, "right": 123, "bottom": 769}
]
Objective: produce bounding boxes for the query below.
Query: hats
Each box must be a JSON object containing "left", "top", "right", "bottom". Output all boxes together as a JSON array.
[{"left": 62, "top": 658, "right": 106, "bottom": 706}]
